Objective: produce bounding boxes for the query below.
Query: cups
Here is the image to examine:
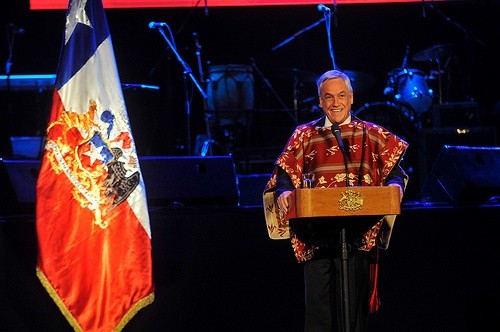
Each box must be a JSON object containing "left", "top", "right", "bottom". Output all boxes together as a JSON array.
[
  {"left": 9, "top": 137, "right": 40, "bottom": 159},
  {"left": 303, "top": 173, "right": 315, "bottom": 188}
]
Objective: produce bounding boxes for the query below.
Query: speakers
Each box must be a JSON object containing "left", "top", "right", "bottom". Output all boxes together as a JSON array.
[
  {"left": 238, "top": 173, "right": 273, "bottom": 208},
  {"left": 421, "top": 145, "right": 500, "bottom": 204},
  {"left": 138, "top": 155, "right": 241, "bottom": 208},
  {"left": 0, "top": 158, "right": 41, "bottom": 217}
]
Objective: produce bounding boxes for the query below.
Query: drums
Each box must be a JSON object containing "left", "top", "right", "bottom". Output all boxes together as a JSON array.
[
  {"left": 386, "top": 67, "right": 436, "bottom": 110},
  {"left": 353, "top": 102, "right": 413, "bottom": 137},
  {"left": 206, "top": 63, "right": 256, "bottom": 146}
]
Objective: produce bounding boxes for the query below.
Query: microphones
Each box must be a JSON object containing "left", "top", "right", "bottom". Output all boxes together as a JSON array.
[
  {"left": 10, "top": 22, "right": 25, "bottom": 34},
  {"left": 331, "top": 124, "right": 346, "bottom": 157},
  {"left": 318, "top": 4, "right": 331, "bottom": 12},
  {"left": 149, "top": 21, "right": 167, "bottom": 28}
]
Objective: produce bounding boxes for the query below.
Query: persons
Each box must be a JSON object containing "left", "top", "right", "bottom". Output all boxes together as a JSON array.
[{"left": 263, "top": 70, "right": 409, "bottom": 332}]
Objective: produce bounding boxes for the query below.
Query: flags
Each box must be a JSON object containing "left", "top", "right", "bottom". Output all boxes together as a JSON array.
[{"left": 35, "top": 0, "right": 155, "bottom": 331}]
